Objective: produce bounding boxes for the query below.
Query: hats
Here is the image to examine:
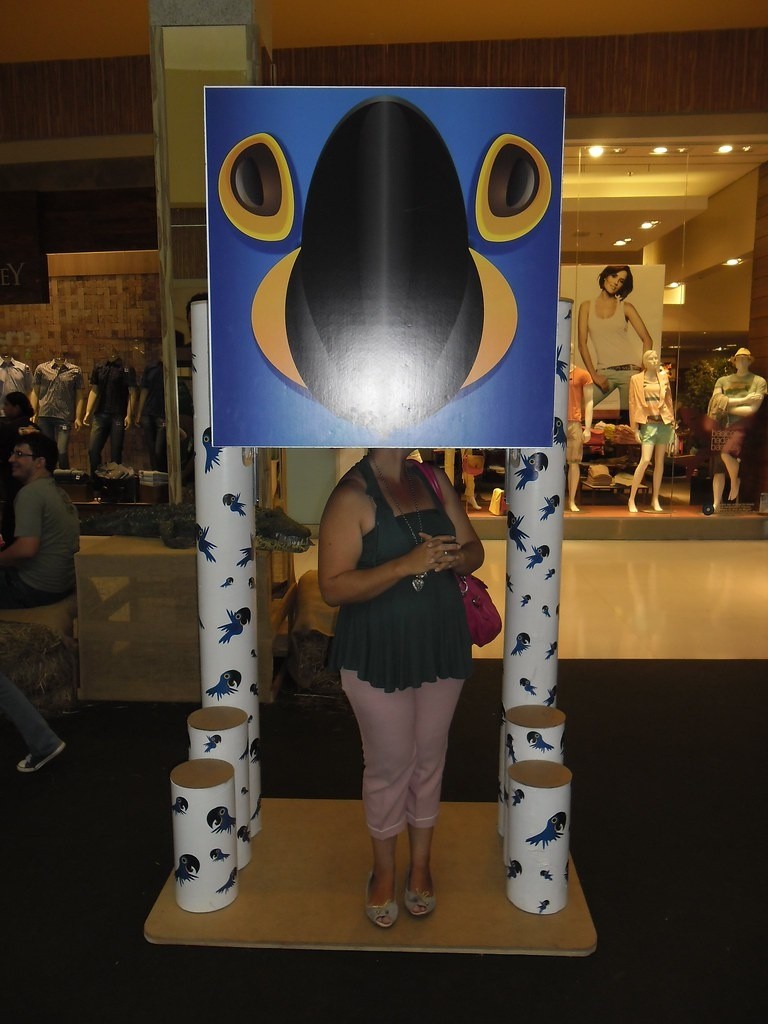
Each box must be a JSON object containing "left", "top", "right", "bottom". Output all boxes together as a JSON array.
[{"left": 730, "top": 348, "right": 754, "bottom": 366}]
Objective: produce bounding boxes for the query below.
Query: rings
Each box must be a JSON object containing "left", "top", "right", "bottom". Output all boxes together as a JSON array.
[{"left": 444, "top": 550, "right": 448, "bottom": 555}]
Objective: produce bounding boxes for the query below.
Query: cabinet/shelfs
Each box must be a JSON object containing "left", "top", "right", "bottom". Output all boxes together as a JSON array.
[{"left": 578, "top": 442, "right": 651, "bottom": 504}]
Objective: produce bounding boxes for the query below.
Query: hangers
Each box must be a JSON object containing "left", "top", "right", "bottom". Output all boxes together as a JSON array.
[{"left": 132, "top": 340, "right": 147, "bottom": 361}]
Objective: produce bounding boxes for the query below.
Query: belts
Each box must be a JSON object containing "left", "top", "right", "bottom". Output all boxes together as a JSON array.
[{"left": 602, "top": 364, "right": 642, "bottom": 372}]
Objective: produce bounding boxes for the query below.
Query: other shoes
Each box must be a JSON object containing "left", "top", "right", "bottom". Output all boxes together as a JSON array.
[{"left": 256, "top": 508, "right": 312, "bottom": 537}]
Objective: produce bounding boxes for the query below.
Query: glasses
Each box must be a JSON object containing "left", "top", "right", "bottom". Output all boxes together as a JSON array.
[{"left": 10, "top": 451, "right": 48, "bottom": 462}]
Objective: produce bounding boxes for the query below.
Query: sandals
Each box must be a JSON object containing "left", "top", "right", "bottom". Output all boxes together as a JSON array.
[
  {"left": 366, "top": 866, "right": 398, "bottom": 927},
  {"left": 403, "top": 864, "right": 436, "bottom": 916}
]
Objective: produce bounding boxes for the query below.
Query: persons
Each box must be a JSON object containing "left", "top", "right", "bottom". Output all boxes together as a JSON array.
[
  {"left": 627, "top": 350, "right": 675, "bottom": 512},
  {"left": 318, "top": 448, "right": 486, "bottom": 928},
  {"left": 577, "top": 266, "right": 654, "bottom": 409},
  {"left": 0, "top": 355, "right": 34, "bottom": 417},
  {"left": 712, "top": 347, "right": 767, "bottom": 509},
  {"left": 29, "top": 357, "right": 85, "bottom": 469},
  {"left": 0, "top": 391, "right": 80, "bottom": 609},
  {"left": 134, "top": 360, "right": 169, "bottom": 474},
  {"left": 567, "top": 343, "right": 593, "bottom": 511},
  {"left": 0, "top": 672, "right": 66, "bottom": 772},
  {"left": 461, "top": 448, "right": 482, "bottom": 510},
  {"left": 83, "top": 354, "right": 136, "bottom": 467}
]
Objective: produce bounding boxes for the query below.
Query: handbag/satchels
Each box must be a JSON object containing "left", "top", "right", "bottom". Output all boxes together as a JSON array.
[
  {"left": 407, "top": 458, "right": 502, "bottom": 648},
  {"left": 489, "top": 488, "right": 506, "bottom": 516},
  {"left": 462, "top": 448, "right": 485, "bottom": 475},
  {"left": 668, "top": 425, "right": 679, "bottom": 456},
  {"left": 710, "top": 414, "right": 742, "bottom": 452}
]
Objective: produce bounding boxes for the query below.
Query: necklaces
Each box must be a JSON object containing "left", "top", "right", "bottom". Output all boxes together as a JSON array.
[{"left": 369, "top": 452, "right": 427, "bottom": 592}]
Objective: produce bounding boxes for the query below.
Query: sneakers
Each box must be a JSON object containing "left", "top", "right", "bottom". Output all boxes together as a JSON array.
[{"left": 17, "top": 736, "right": 66, "bottom": 772}]
